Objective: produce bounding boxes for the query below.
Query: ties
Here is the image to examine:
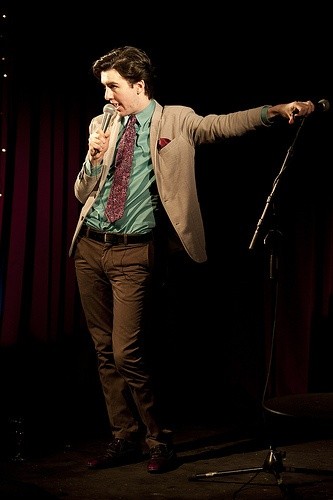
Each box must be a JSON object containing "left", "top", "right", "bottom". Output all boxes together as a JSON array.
[{"left": 103, "top": 115, "right": 137, "bottom": 223}]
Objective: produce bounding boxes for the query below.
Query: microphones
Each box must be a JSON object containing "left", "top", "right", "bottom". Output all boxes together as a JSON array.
[
  {"left": 291, "top": 99, "right": 330, "bottom": 115},
  {"left": 91, "top": 104, "right": 116, "bottom": 156}
]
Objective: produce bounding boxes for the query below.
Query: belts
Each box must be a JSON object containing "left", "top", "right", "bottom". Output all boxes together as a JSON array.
[{"left": 80, "top": 227, "right": 152, "bottom": 244}]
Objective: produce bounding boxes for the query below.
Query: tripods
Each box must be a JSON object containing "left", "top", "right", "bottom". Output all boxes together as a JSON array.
[{"left": 186, "top": 113, "right": 333, "bottom": 495}]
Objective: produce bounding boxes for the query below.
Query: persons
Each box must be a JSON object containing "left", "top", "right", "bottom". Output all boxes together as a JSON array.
[{"left": 69, "top": 47, "right": 314, "bottom": 472}]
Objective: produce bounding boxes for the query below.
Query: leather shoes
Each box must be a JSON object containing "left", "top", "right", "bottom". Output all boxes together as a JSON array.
[
  {"left": 146, "top": 445, "right": 176, "bottom": 472},
  {"left": 88, "top": 440, "right": 139, "bottom": 469}
]
